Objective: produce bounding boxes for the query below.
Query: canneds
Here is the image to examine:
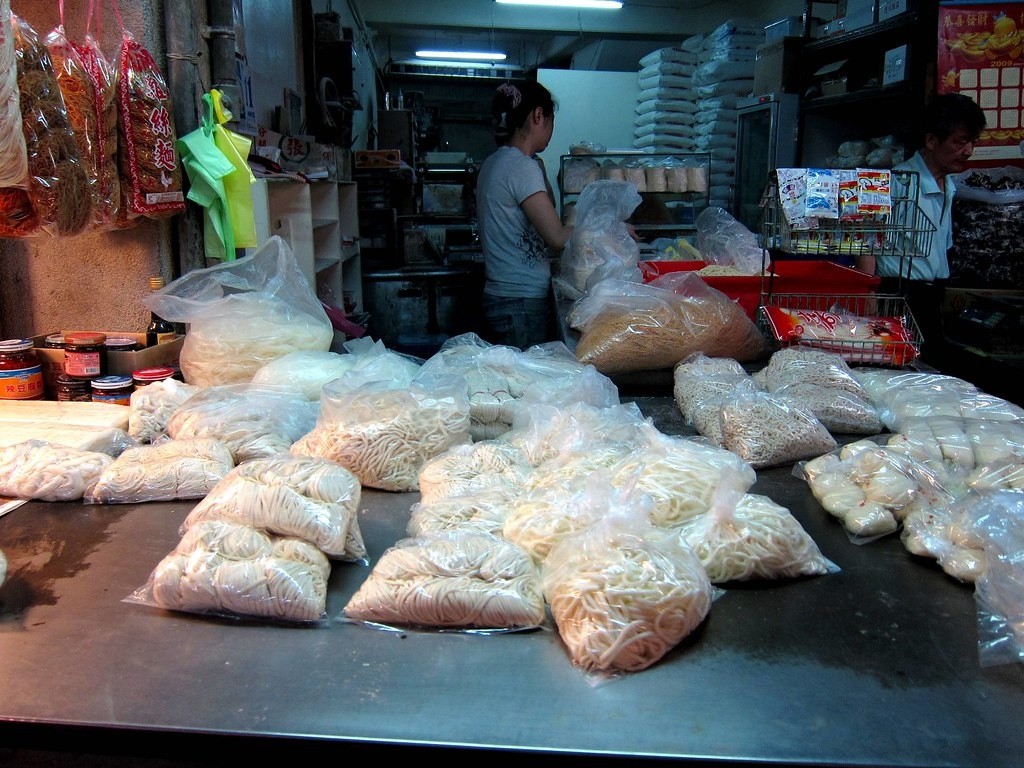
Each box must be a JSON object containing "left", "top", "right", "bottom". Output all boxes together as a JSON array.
[{"left": 0, "top": 332, "right": 174, "bottom": 407}]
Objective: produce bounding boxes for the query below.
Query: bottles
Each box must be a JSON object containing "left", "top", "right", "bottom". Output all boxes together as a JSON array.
[{"left": 146, "top": 276, "right": 177, "bottom": 348}]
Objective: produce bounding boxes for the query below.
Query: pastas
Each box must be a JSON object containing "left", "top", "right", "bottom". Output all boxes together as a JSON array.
[
  {"left": 87, "top": 254, "right": 887, "bottom": 675},
  {"left": 0, "top": 437, "right": 115, "bottom": 502}
]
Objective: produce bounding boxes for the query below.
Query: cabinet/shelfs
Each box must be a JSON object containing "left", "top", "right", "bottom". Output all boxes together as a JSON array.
[
  {"left": 243, "top": 178, "right": 367, "bottom": 336},
  {"left": 557, "top": 151, "right": 712, "bottom": 243},
  {"left": 795, "top": 12, "right": 939, "bottom": 169},
  {"left": 751, "top": 169, "right": 938, "bottom": 371}
]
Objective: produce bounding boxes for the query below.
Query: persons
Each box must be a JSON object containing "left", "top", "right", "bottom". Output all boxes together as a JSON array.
[
  {"left": 474, "top": 79, "right": 637, "bottom": 352},
  {"left": 557, "top": 159, "right": 679, "bottom": 242},
  {"left": 857, "top": 93, "right": 986, "bottom": 346}
]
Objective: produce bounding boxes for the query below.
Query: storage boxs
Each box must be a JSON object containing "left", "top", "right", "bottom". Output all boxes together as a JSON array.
[
  {"left": 754, "top": 36, "right": 815, "bottom": 96},
  {"left": 26, "top": 329, "right": 186, "bottom": 395},
  {"left": 814, "top": 0, "right": 911, "bottom": 40},
  {"left": 763, "top": 16, "right": 818, "bottom": 43}
]
